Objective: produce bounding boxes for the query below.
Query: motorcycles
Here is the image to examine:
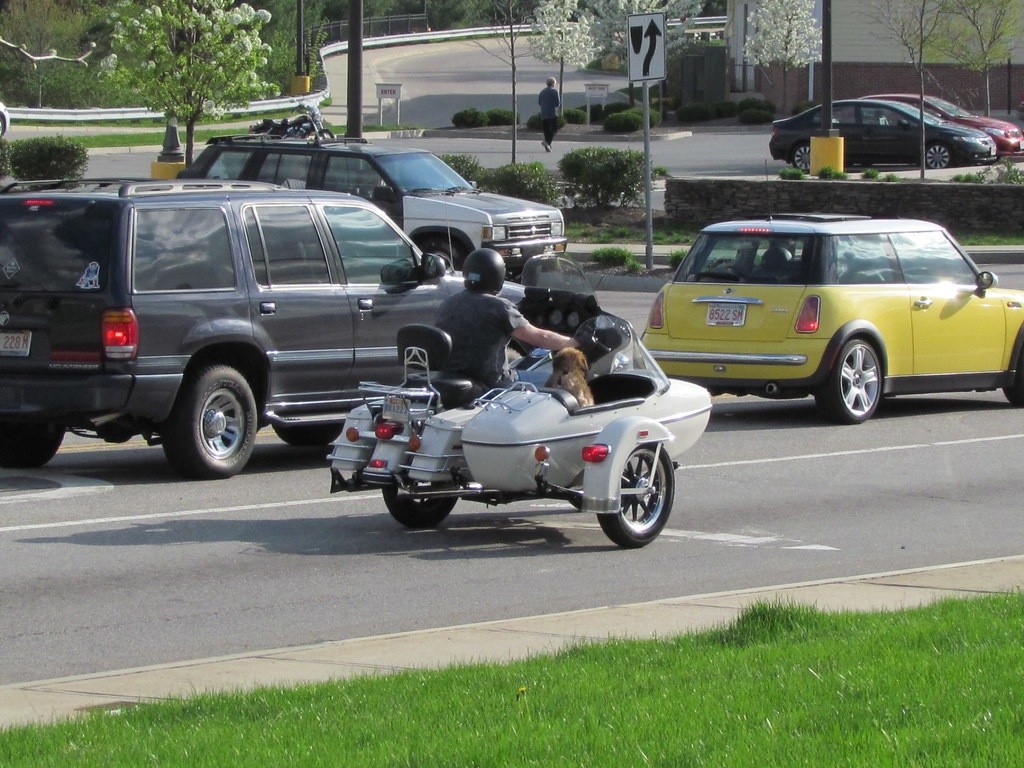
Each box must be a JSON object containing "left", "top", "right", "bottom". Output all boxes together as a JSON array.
[
  {"left": 248, "top": 98, "right": 334, "bottom": 141},
  {"left": 324, "top": 255, "right": 710, "bottom": 548}
]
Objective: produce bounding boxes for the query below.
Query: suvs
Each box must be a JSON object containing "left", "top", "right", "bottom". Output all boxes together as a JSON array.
[
  {"left": 0, "top": 179, "right": 525, "bottom": 480},
  {"left": 637, "top": 215, "right": 1024, "bottom": 425},
  {"left": 176, "top": 135, "right": 568, "bottom": 281}
]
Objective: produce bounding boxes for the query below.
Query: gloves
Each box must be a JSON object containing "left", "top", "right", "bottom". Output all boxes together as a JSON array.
[{"left": 578, "top": 334, "right": 598, "bottom": 352}]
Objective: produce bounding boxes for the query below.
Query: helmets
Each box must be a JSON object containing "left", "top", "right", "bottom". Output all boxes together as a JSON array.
[{"left": 463, "top": 249, "right": 506, "bottom": 289}]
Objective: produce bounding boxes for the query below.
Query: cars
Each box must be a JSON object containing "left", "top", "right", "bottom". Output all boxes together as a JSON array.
[
  {"left": 860, "top": 93, "right": 1024, "bottom": 161},
  {"left": 769, "top": 99, "right": 998, "bottom": 173}
]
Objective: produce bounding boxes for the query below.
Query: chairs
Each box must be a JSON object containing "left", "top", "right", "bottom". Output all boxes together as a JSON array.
[
  {"left": 397, "top": 324, "right": 482, "bottom": 405},
  {"left": 761, "top": 247, "right": 792, "bottom": 284},
  {"left": 508, "top": 383, "right": 578, "bottom": 412},
  {"left": 874, "top": 109, "right": 889, "bottom": 125}
]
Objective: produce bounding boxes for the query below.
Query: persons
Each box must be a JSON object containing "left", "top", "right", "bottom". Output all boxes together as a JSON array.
[
  {"left": 434, "top": 248, "right": 581, "bottom": 388},
  {"left": 539, "top": 77, "right": 560, "bottom": 154}
]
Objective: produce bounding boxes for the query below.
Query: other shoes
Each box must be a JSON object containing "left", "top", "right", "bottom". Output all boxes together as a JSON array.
[{"left": 541, "top": 142, "right": 552, "bottom": 152}]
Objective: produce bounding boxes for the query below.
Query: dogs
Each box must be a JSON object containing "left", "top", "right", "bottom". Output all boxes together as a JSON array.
[{"left": 545, "top": 347, "right": 595, "bottom": 408}]
